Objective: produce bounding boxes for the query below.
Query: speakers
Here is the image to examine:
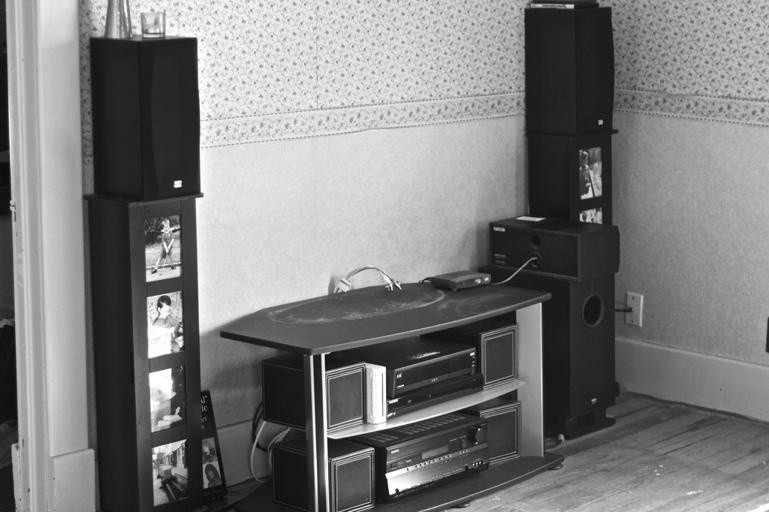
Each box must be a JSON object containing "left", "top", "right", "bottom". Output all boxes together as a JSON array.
[
  {"left": 260, "top": 353, "right": 368, "bottom": 433},
  {"left": 271, "top": 441, "right": 376, "bottom": 512},
  {"left": 477, "top": 265, "right": 620, "bottom": 441},
  {"left": 90, "top": 33, "right": 200, "bottom": 200},
  {"left": 524, "top": 7, "right": 614, "bottom": 134},
  {"left": 457, "top": 320, "right": 516, "bottom": 385},
  {"left": 465, "top": 395, "right": 521, "bottom": 465}
]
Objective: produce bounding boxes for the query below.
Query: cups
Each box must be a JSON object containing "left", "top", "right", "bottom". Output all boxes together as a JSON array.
[{"left": 139, "top": 11, "right": 166, "bottom": 39}]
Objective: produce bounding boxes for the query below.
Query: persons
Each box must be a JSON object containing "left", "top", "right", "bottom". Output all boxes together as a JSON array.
[
  {"left": 150, "top": 298, "right": 176, "bottom": 356},
  {"left": 204, "top": 464, "right": 225, "bottom": 486},
  {"left": 202, "top": 439, "right": 219, "bottom": 463},
  {"left": 593, "top": 148, "right": 603, "bottom": 197},
  {"left": 578, "top": 151, "right": 594, "bottom": 200},
  {"left": 152, "top": 216, "right": 176, "bottom": 272}
]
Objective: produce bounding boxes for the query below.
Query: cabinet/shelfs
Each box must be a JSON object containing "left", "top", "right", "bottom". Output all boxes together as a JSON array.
[{"left": 304, "top": 302, "right": 564, "bottom": 512}]
